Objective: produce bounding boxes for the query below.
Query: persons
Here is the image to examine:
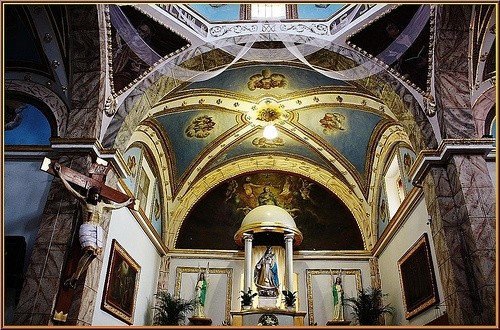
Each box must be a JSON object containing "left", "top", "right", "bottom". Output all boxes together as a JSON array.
[
  {"left": 333, "top": 277, "right": 344, "bottom": 320},
  {"left": 52, "top": 161, "right": 133, "bottom": 290},
  {"left": 193, "top": 272, "right": 207, "bottom": 316}
]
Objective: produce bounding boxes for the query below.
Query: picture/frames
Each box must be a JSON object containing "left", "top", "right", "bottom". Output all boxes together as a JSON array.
[
  {"left": 397, "top": 233, "right": 439, "bottom": 320},
  {"left": 100, "top": 238, "right": 143, "bottom": 325}
]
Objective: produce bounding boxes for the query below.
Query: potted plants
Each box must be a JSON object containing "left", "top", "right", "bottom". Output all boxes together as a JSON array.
[
  {"left": 281, "top": 290, "right": 298, "bottom": 311},
  {"left": 236, "top": 287, "right": 258, "bottom": 311}
]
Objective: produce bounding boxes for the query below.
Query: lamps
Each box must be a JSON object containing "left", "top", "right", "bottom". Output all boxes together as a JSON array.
[{"left": 264, "top": 121, "right": 278, "bottom": 140}]
